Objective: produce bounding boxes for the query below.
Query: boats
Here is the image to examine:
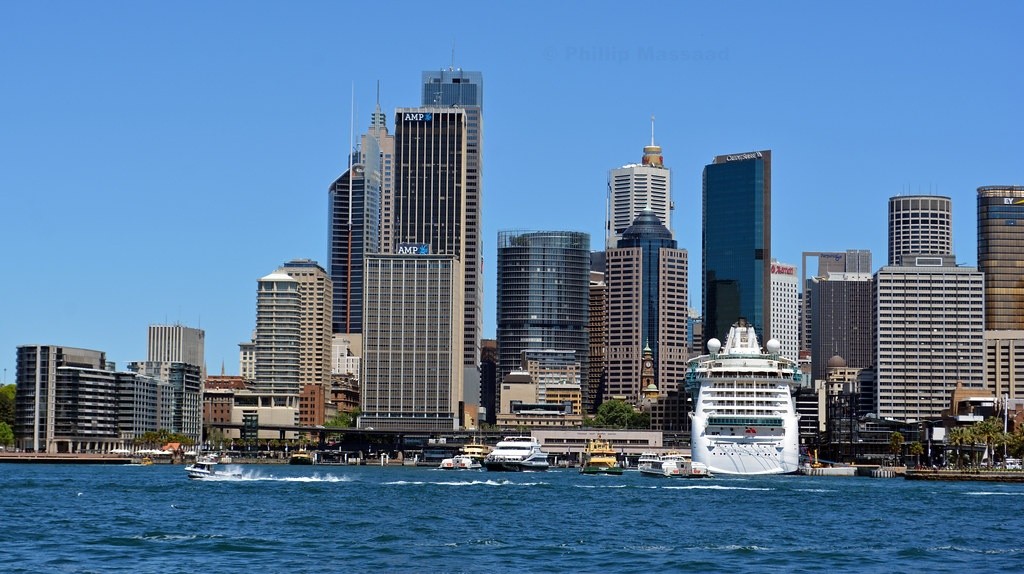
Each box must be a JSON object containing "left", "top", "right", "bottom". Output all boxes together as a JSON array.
[
  {"left": 438, "top": 458, "right": 482, "bottom": 469},
  {"left": 288, "top": 448, "right": 313, "bottom": 465},
  {"left": 416, "top": 449, "right": 455, "bottom": 467},
  {"left": 184, "top": 462, "right": 243, "bottom": 480},
  {"left": 484, "top": 435, "right": 550, "bottom": 471},
  {"left": 578, "top": 434, "right": 623, "bottom": 475},
  {"left": 130, "top": 450, "right": 175, "bottom": 464},
  {"left": 638, "top": 452, "right": 716, "bottom": 478},
  {"left": 141, "top": 458, "right": 153, "bottom": 465},
  {"left": 684, "top": 318, "right": 802, "bottom": 475},
  {"left": 461, "top": 444, "right": 492, "bottom": 467}
]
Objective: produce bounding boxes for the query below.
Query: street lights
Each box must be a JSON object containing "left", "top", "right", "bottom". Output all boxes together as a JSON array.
[{"left": 921, "top": 397, "right": 938, "bottom": 470}]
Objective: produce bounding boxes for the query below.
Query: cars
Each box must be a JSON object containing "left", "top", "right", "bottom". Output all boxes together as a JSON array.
[{"left": 996, "top": 461, "right": 1016, "bottom": 470}]
